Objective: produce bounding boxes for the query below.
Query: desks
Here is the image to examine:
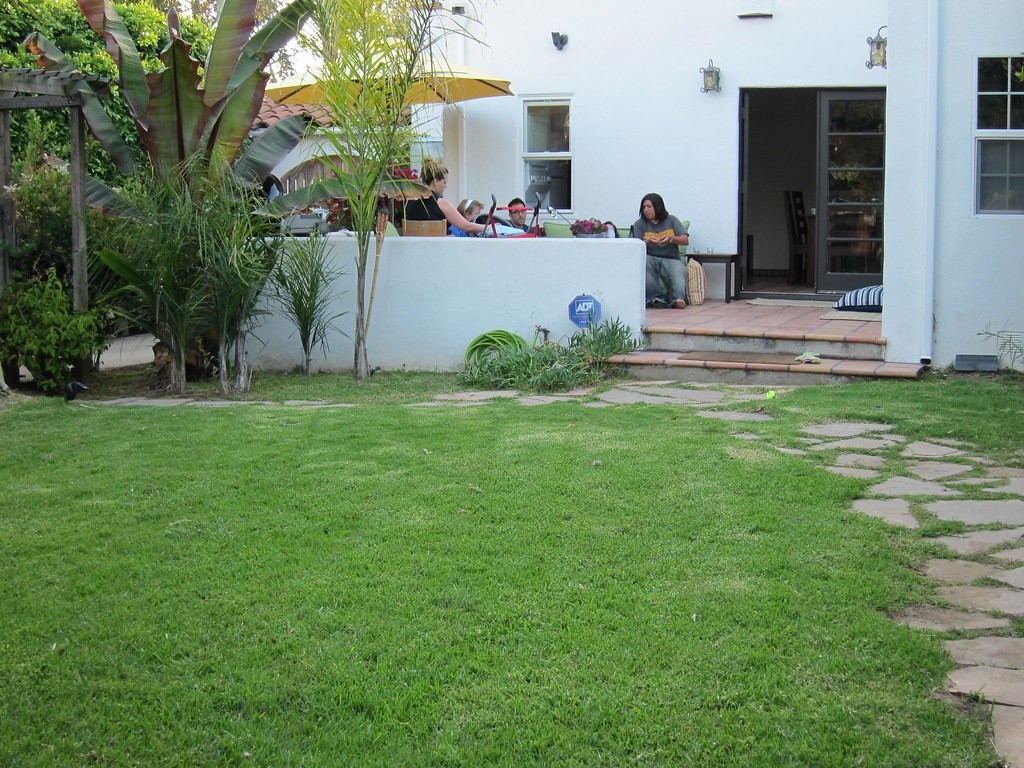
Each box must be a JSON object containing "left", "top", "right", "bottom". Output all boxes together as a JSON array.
[{"left": 685, "top": 253, "right": 741, "bottom": 303}]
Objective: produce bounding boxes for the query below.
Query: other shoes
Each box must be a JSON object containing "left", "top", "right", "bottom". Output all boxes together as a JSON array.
[
  {"left": 676, "top": 300, "right": 685, "bottom": 309},
  {"left": 652, "top": 300, "right": 661, "bottom": 307}
]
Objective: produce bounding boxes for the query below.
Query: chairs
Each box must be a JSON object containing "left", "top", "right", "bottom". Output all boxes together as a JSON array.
[
  {"left": 783, "top": 190, "right": 809, "bottom": 285},
  {"left": 401, "top": 219, "right": 447, "bottom": 237}
]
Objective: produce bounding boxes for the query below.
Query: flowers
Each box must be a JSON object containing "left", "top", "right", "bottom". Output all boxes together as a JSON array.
[{"left": 570, "top": 217, "right": 608, "bottom": 236}]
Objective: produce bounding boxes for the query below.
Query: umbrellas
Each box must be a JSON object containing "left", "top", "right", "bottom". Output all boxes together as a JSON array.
[{"left": 264, "top": 37, "right": 514, "bottom": 222}]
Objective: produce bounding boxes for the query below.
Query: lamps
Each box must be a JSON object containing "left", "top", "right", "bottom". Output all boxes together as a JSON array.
[
  {"left": 699, "top": 59, "right": 721, "bottom": 93},
  {"left": 865, "top": 26, "right": 887, "bottom": 70}
]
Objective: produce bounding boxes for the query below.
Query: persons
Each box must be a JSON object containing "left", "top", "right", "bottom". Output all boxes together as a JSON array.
[
  {"left": 603, "top": 221, "right": 619, "bottom": 238},
  {"left": 422, "top": 158, "right": 501, "bottom": 236},
  {"left": 634, "top": 193, "right": 689, "bottom": 309},
  {"left": 507, "top": 199, "right": 538, "bottom": 237},
  {"left": 449, "top": 199, "right": 484, "bottom": 237}
]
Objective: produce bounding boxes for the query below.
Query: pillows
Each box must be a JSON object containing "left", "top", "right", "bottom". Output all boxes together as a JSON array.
[{"left": 831, "top": 284, "right": 883, "bottom": 312}]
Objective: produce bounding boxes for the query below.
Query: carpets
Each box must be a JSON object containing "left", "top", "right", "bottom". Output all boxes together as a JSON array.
[{"left": 820, "top": 311, "right": 882, "bottom": 322}]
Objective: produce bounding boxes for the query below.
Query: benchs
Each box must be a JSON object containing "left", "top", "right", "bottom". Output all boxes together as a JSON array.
[{"left": 543, "top": 219, "right": 689, "bottom": 308}]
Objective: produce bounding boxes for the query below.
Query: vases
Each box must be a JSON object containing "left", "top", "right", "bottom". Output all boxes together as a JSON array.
[{"left": 577, "top": 233, "right": 603, "bottom": 238}]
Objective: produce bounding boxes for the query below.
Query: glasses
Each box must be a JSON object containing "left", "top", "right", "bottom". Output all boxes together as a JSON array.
[{"left": 511, "top": 210, "right": 527, "bottom": 215}]
